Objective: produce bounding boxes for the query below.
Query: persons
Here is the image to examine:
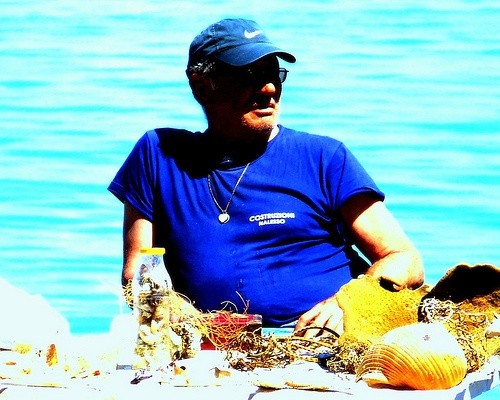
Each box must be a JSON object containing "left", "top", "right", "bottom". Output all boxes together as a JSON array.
[{"left": 107, "top": 16, "right": 426, "bottom": 342}]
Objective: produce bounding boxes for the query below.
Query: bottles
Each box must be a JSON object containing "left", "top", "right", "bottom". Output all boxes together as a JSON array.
[{"left": 131, "top": 247, "right": 173, "bottom": 370}]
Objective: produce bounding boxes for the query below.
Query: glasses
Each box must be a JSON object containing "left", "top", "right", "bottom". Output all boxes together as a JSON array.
[{"left": 204, "top": 68, "right": 290, "bottom": 87}]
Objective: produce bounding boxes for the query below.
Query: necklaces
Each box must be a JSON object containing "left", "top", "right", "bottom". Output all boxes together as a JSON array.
[{"left": 204, "top": 130, "right": 270, "bottom": 224}]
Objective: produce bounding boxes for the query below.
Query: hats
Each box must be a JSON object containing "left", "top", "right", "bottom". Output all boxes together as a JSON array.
[{"left": 186, "top": 19, "right": 298, "bottom": 64}]
